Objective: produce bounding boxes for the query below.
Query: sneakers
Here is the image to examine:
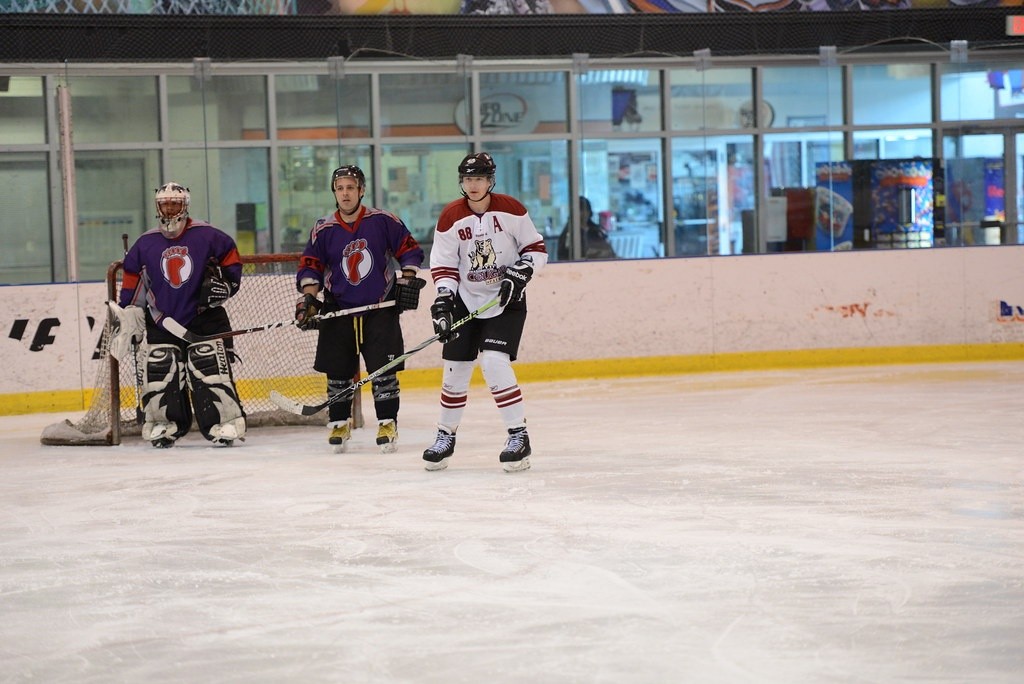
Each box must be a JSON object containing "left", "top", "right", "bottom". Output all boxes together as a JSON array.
[
  {"left": 423, "top": 424, "right": 455, "bottom": 469},
  {"left": 500, "top": 426, "right": 532, "bottom": 471},
  {"left": 376, "top": 419, "right": 398, "bottom": 452},
  {"left": 328, "top": 418, "right": 351, "bottom": 454}
]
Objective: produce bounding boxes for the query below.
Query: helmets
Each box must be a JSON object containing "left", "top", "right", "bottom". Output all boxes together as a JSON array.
[
  {"left": 458, "top": 152, "right": 497, "bottom": 178},
  {"left": 331, "top": 165, "right": 367, "bottom": 199},
  {"left": 154, "top": 182, "right": 191, "bottom": 239}
]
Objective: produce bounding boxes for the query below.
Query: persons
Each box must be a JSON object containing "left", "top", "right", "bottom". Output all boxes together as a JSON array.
[
  {"left": 294, "top": 165, "right": 426, "bottom": 454},
  {"left": 422, "top": 151, "right": 549, "bottom": 472},
  {"left": 556, "top": 196, "right": 618, "bottom": 261},
  {"left": 117, "top": 182, "right": 248, "bottom": 449}
]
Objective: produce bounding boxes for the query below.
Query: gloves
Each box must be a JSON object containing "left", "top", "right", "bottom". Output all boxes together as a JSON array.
[
  {"left": 394, "top": 277, "right": 426, "bottom": 316},
  {"left": 296, "top": 294, "right": 323, "bottom": 330},
  {"left": 430, "top": 295, "right": 460, "bottom": 343},
  {"left": 500, "top": 261, "right": 533, "bottom": 306}
]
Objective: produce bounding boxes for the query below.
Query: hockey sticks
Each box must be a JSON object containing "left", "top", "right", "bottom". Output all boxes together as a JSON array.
[
  {"left": 268, "top": 296, "right": 501, "bottom": 419},
  {"left": 161, "top": 299, "right": 397, "bottom": 344},
  {"left": 122, "top": 233, "right": 146, "bottom": 427}
]
